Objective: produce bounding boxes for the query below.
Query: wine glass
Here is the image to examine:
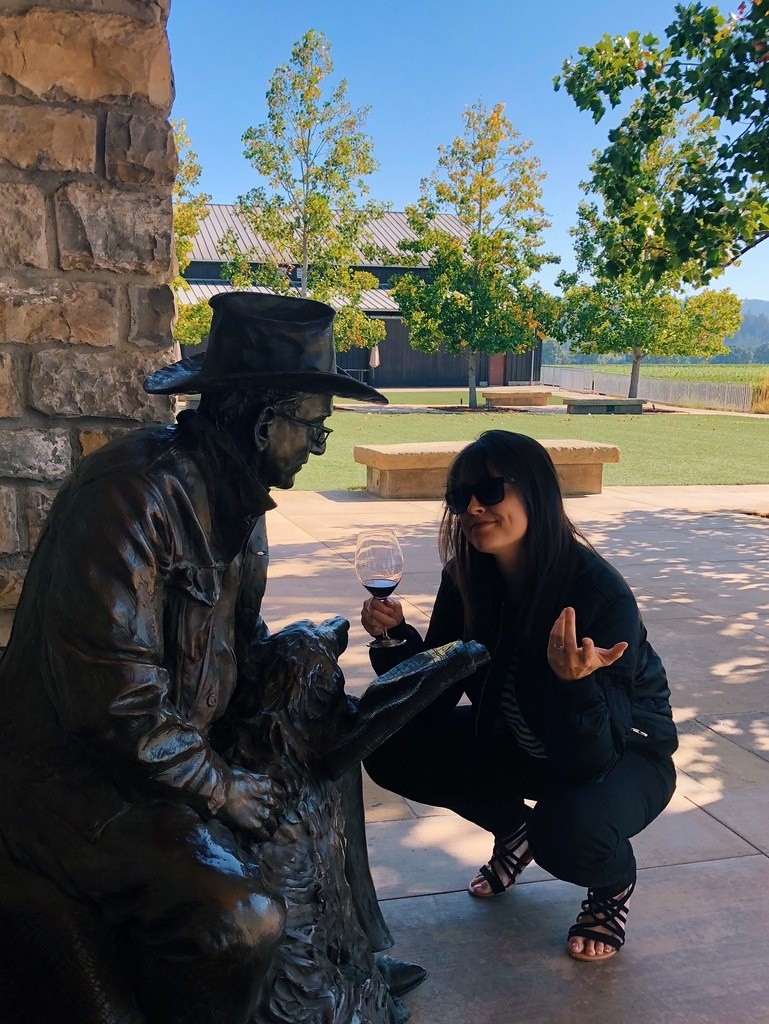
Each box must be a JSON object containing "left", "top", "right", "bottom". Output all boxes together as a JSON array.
[{"left": 354, "top": 528, "right": 408, "bottom": 647}]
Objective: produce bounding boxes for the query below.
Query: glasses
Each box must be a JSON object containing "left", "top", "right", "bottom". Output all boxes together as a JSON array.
[{"left": 444, "top": 476, "right": 517, "bottom": 514}]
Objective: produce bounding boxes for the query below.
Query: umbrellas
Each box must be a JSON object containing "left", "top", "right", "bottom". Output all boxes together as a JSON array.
[{"left": 368, "top": 346, "right": 381, "bottom": 383}]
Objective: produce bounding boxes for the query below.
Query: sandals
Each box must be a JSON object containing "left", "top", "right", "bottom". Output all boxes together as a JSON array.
[
  {"left": 567, "top": 883, "right": 634, "bottom": 960},
  {"left": 468, "top": 823, "right": 533, "bottom": 896}
]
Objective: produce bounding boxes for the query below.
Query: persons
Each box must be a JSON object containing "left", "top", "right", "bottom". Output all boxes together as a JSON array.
[
  {"left": 360, "top": 430, "right": 679, "bottom": 962},
  {"left": 0, "top": 292, "right": 428, "bottom": 1024}
]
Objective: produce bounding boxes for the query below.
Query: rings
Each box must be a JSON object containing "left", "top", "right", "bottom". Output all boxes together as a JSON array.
[{"left": 553, "top": 642, "right": 564, "bottom": 650}]
